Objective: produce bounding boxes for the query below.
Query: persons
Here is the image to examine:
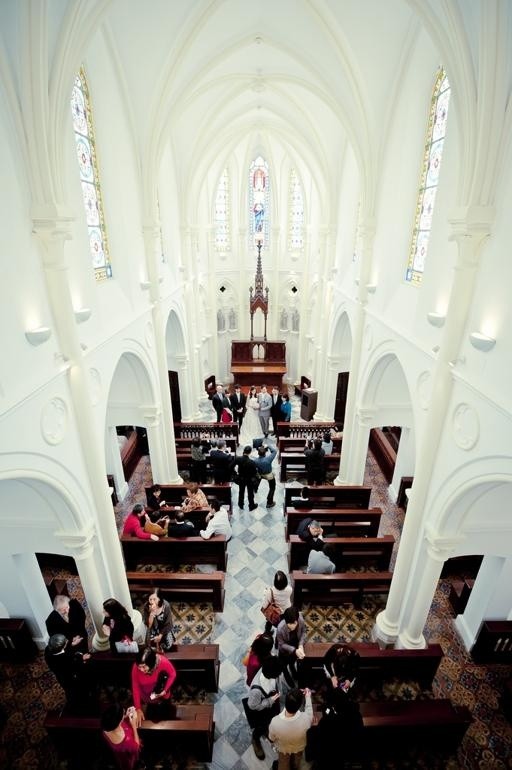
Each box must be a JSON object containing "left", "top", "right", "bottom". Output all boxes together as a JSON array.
[
  {"left": 297, "top": 517, "right": 337, "bottom": 574},
  {"left": 122, "top": 477, "right": 232, "bottom": 543},
  {"left": 247, "top": 571, "right": 361, "bottom": 769},
  {"left": 304, "top": 433, "right": 333, "bottom": 485},
  {"left": 46, "top": 588, "right": 177, "bottom": 767},
  {"left": 212, "top": 384, "right": 291, "bottom": 444},
  {"left": 191, "top": 434, "right": 277, "bottom": 511}
]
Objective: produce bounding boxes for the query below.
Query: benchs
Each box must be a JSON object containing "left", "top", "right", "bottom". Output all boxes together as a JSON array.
[
  {"left": 41, "top": 701, "right": 216, "bottom": 763},
  {"left": 293, "top": 375, "right": 312, "bottom": 397},
  {"left": 205, "top": 374, "right": 225, "bottom": 400},
  {"left": 305, "top": 696, "right": 470, "bottom": 761},
  {"left": 120, "top": 421, "right": 240, "bottom": 573},
  {"left": 275, "top": 421, "right": 396, "bottom": 573},
  {"left": 449, "top": 579, "right": 477, "bottom": 615},
  {"left": 127, "top": 571, "right": 228, "bottom": 613},
  {"left": 76, "top": 642, "right": 222, "bottom": 695},
  {"left": 297, "top": 640, "right": 445, "bottom": 690},
  {"left": 291, "top": 567, "right": 394, "bottom": 611}
]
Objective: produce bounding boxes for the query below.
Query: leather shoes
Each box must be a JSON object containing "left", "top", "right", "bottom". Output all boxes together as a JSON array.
[
  {"left": 264, "top": 432, "right": 277, "bottom": 438},
  {"left": 266, "top": 502, "right": 275, "bottom": 507},
  {"left": 240, "top": 503, "right": 258, "bottom": 511}
]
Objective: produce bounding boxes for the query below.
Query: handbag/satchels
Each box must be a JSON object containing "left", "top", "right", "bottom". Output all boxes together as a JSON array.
[
  {"left": 243, "top": 648, "right": 251, "bottom": 665},
  {"left": 261, "top": 601, "right": 281, "bottom": 625},
  {"left": 154, "top": 671, "right": 168, "bottom": 694},
  {"left": 242, "top": 690, "right": 280, "bottom": 729},
  {"left": 115, "top": 641, "right": 139, "bottom": 653}
]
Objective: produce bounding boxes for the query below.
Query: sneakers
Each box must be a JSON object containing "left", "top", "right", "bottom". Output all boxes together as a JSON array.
[
  {"left": 272, "top": 760, "right": 278, "bottom": 770},
  {"left": 252, "top": 738, "right": 265, "bottom": 760},
  {"left": 284, "top": 664, "right": 297, "bottom": 688}
]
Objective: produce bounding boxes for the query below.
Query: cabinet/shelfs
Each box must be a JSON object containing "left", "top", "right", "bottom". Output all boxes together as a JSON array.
[
  {"left": 471, "top": 620, "right": 512, "bottom": 666},
  {"left": 0, "top": 618, "right": 40, "bottom": 663}
]
